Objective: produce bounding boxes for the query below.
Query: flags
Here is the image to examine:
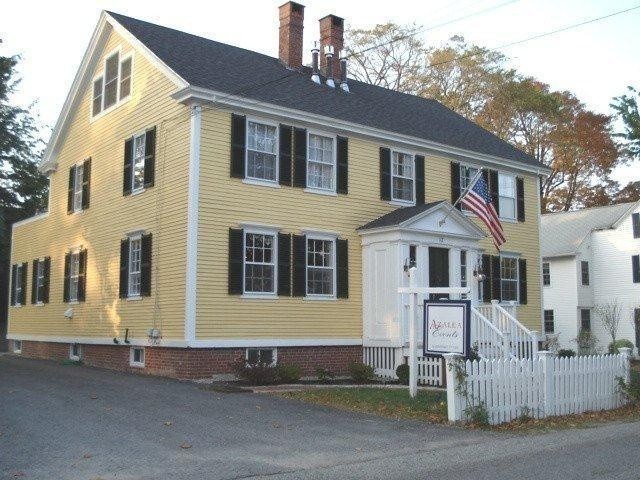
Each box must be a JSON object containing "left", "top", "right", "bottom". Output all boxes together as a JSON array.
[{"left": 457, "top": 172, "right": 507, "bottom": 254}]
